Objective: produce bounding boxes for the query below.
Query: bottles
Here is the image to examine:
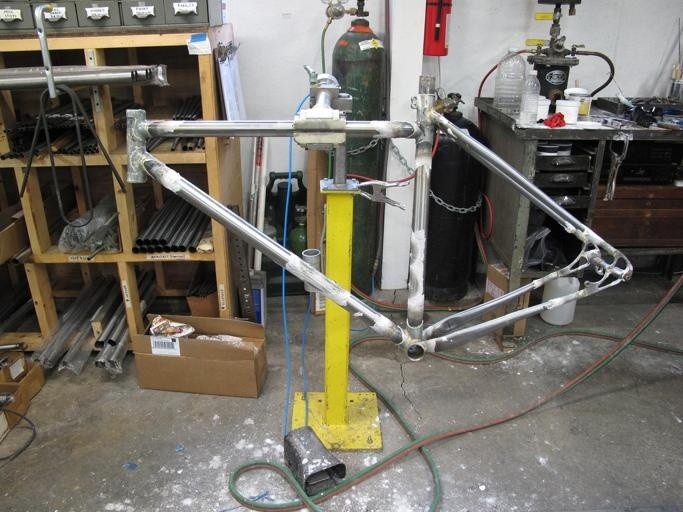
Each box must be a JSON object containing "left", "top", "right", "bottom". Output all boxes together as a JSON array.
[{"left": 519, "top": 69, "right": 542, "bottom": 125}]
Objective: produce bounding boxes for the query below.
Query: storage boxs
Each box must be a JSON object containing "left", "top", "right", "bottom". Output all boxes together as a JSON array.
[{"left": 127, "top": 311, "right": 270, "bottom": 401}]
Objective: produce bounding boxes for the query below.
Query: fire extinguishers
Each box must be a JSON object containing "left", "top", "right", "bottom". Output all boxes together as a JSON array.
[{"left": 422, "top": 0, "right": 453, "bottom": 56}]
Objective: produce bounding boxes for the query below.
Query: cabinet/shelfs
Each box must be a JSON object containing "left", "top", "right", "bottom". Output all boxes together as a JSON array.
[{"left": 0, "top": 23, "right": 252, "bottom": 358}]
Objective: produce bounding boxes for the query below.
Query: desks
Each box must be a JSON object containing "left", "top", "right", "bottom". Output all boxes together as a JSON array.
[{"left": 476, "top": 97, "right": 683, "bottom": 355}]
